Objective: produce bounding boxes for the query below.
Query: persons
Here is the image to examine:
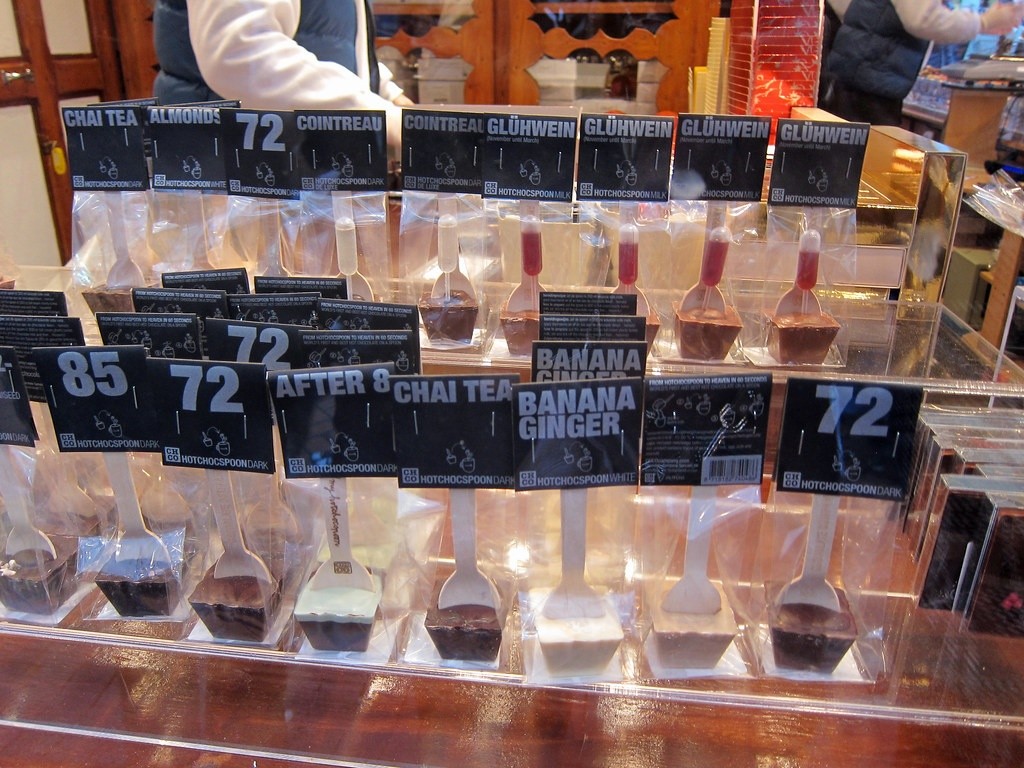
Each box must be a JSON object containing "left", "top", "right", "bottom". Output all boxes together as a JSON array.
[
  {"left": 153, "top": 0, "right": 411, "bottom": 151},
  {"left": 826, "top": 0, "right": 1024, "bottom": 127},
  {"left": 819, "top": 0, "right": 852, "bottom": 107}
]
